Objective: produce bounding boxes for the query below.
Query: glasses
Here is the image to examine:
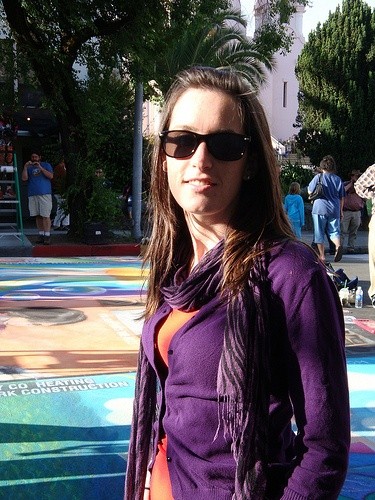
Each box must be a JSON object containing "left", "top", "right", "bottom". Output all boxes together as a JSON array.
[{"left": 158, "top": 130, "right": 252, "bottom": 162}]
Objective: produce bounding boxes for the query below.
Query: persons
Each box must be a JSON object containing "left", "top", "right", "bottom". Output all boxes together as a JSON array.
[
  {"left": 284, "top": 183, "right": 305, "bottom": 244},
  {"left": 22, "top": 151, "right": 55, "bottom": 246},
  {"left": 339, "top": 169, "right": 365, "bottom": 254},
  {"left": 354, "top": 164, "right": 375, "bottom": 309},
  {"left": 52, "top": 158, "right": 72, "bottom": 232},
  {"left": 306, "top": 155, "right": 343, "bottom": 265},
  {"left": 123, "top": 65, "right": 351, "bottom": 500},
  {"left": 120, "top": 181, "right": 133, "bottom": 231},
  {"left": 311, "top": 168, "right": 336, "bottom": 257}
]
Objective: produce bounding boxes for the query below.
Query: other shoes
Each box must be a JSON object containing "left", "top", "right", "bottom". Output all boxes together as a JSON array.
[
  {"left": 36, "top": 223, "right": 70, "bottom": 245},
  {"left": 345, "top": 246, "right": 361, "bottom": 254}
]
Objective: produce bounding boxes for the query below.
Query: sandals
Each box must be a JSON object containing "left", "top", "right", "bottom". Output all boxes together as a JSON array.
[{"left": 334, "top": 245, "right": 343, "bottom": 262}]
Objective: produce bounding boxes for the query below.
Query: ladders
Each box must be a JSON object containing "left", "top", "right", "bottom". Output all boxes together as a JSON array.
[{"left": 0, "top": 149, "right": 25, "bottom": 247}]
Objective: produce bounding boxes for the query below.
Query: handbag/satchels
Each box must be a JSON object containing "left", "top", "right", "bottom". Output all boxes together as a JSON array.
[{"left": 308, "top": 184, "right": 325, "bottom": 200}]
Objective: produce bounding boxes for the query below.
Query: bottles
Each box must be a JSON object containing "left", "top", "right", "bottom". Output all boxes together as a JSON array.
[
  {"left": 355, "top": 286, "right": 363, "bottom": 308},
  {"left": 339, "top": 287, "right": 349, "bottom": 307}
]
